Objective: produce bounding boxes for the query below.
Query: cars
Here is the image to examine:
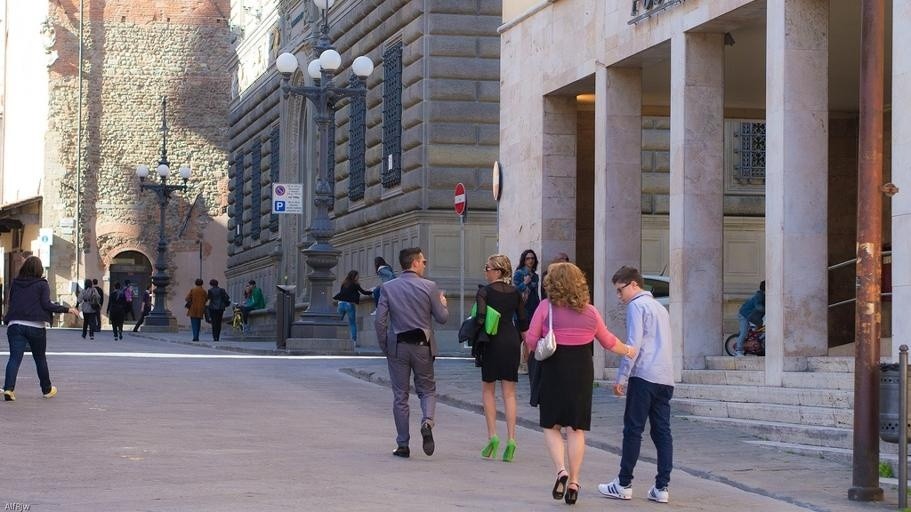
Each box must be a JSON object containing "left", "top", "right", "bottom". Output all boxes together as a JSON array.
[{"left": 641, "top": 270, "right": 672, "bottom": 311}]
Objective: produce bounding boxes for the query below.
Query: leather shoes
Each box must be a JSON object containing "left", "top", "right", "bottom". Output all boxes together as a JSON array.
[
  {"left": 420, "top": 423, "right": 434, "bottom": 455},
  {"left": 392, "top": 447, "right": 411, "bottom": 458}
]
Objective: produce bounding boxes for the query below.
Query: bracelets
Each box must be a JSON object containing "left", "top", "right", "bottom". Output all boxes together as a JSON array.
[{"left": 625, "top": 343, "right": 631, "bottom": 356}]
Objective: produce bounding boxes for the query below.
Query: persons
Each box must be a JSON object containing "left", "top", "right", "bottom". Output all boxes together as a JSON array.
[
  {"left": 241, "top": 279, "right": 267, "bottom": 331},
  {"left": 598, "top": 265, "right": 676, "bottom": 502},
  {"left": 376, "top": 248, "right": 449, "bottom": 457},
  {"left": 186, "top": 278, "right": 210, "bottom": 341},
  {"left": 208, "top": 279, "right": 232, "bottom": 341},
  {"left": 460, "top": 255, "right": 531, "bottom": 463},
  {"left": 3, "top": 255, "right": 82, "bottom": 400},
  {"left": 333, "top": 269, "right": 375, "bottom": 347},
  {"left": 228, "top": 284, "right": 252, "bottom": 325},
  {"left": 736, "top": 279, "right": 765, "bottom": 357},
  {"left": 77, "top": 279, "right": 153, "bottom": 342},
  {"left": 542, "top": 252, "right": 570, "bottom": 300},
  {"left": 513, "top": 249, "right": 543, "bottom": 375},
  {"left": 370, "top": 257, "right": 397, "bottom": 316},
  {"left": 523, "top": 261, "right": 637, "bottom": 505}
]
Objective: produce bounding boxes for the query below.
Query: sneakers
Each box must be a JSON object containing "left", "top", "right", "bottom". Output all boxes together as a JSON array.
[
  {"left": 192, "top": 337, "right": 220, "bottom": 342},
  {"left": 646, "top": 483, "right": 669, "bottom": 503},
  {"left": 82, "top": 329, "right": 138, "bottom": 341},
  {"left": 43, "top": 387, "right": 57, "bottom": 398},
  {"left": 3, "top": 391, "right": 15, "bottom": 403},
  {"left": 598, "top": 478, "right": 633, "bottom": 500}
]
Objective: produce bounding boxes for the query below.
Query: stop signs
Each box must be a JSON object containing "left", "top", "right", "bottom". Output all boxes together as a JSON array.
[{"left": 454, "top": 181, "right": 467, "bottom": 216}]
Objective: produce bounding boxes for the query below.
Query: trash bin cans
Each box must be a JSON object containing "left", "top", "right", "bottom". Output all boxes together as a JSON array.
[{"left": 879, "top": 363, "right": 911, "bottom": 509}]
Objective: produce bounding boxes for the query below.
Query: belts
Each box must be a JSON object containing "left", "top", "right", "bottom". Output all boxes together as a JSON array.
[{"left": 398, "top": 339, "right": 429, "bottom": 346}]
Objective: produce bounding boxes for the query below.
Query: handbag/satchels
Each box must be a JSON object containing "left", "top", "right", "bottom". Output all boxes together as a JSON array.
[
  {"left": 185, "top": 300, "right": 193, "bottom": 309},
  {"left": 89, "top": 292, "right": 101, "bottom": 310},
  {"left": 534, "top": 302, "right": 558, "bottom": 361},
  {"left": 204, "top": 305, "right": 213, "bottom": 323},
  {"left": 469, "top": 299, "right": 502, "bottom": 338}
]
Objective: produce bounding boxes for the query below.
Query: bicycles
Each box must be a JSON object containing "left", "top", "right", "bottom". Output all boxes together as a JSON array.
[
  {"left": 229, "top": 299, "right": 246, "bottom": 335},
  {"left": 722, "top": 315, "right": 766, "bottom": 358}
]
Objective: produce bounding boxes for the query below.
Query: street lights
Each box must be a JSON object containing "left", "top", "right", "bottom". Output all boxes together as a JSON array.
[
  {"left": 133, "top": 148, "right": 194, "bottom": 333},
  {"left": 275, "top": 13, "right": 374, "bottom": 353}
]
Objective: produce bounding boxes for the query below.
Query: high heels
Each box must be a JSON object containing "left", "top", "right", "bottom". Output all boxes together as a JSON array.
[
  {"left": 502, "top": 439, "right": 516, "bottom": 461},
  {"left": 481, "top": 435, "right": 500, "bottom": 459},
  {"left": 565, "top": 481, "right": 580, "bottom": 504},
  {"left": 552, "top": 470, "right": 568, "bottom": 499}
]
